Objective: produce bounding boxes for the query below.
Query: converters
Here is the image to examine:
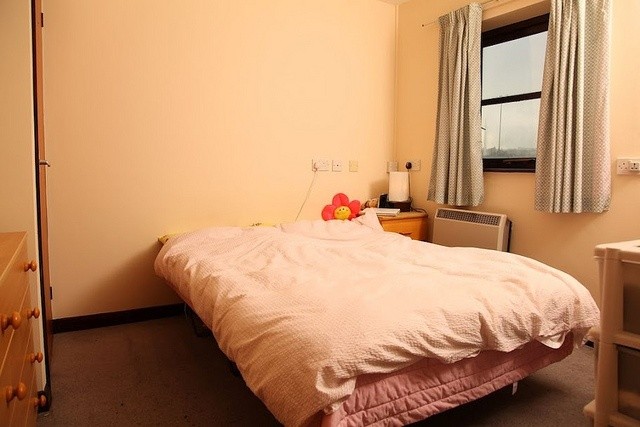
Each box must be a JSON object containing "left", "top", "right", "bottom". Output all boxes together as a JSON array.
[{"left": 405, "top": 162, "right": 411, "bottom": 169}]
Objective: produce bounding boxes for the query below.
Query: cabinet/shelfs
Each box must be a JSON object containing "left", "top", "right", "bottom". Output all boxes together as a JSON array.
[
  {"left": 584, "top": 241, "right": 640, "bottom": 427},
  {"left": 0, "top": 0, "right": 54, "bottom": 412},
  {"left": 0, "top": 231, "right": 46, "bottom": 427}
]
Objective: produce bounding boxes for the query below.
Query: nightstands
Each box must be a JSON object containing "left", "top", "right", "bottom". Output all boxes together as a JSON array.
[{"left": 376, "top": 211, "right": 429, "bottom": 241}]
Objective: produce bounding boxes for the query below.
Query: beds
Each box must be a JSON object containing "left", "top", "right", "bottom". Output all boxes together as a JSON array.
[{"left": 149, "top": 220, "right": 602, "bottom": 426}]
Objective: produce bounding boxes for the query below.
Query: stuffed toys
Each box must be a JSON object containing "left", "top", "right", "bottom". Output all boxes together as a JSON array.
[{"left": 322, "top": 193, "right": 360, "bottom": 222}]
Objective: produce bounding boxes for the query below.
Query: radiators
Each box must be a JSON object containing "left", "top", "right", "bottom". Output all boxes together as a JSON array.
[{"left": 432, "top": 206, "right": 511, "bottom": 251}]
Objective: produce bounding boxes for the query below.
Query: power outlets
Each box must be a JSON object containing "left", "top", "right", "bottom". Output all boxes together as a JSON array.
[
  {"left": 615, "top": 158, "right": 640, "bottom": 175},
  {"left": 332, "top": 159, "right": 344, "bottom": 172},
  {"left": 313, "top": 158, "right": 330, "bottom": 171},
  {"left": 406, "top": 160, "right": 421, "bottom": 171}
]
